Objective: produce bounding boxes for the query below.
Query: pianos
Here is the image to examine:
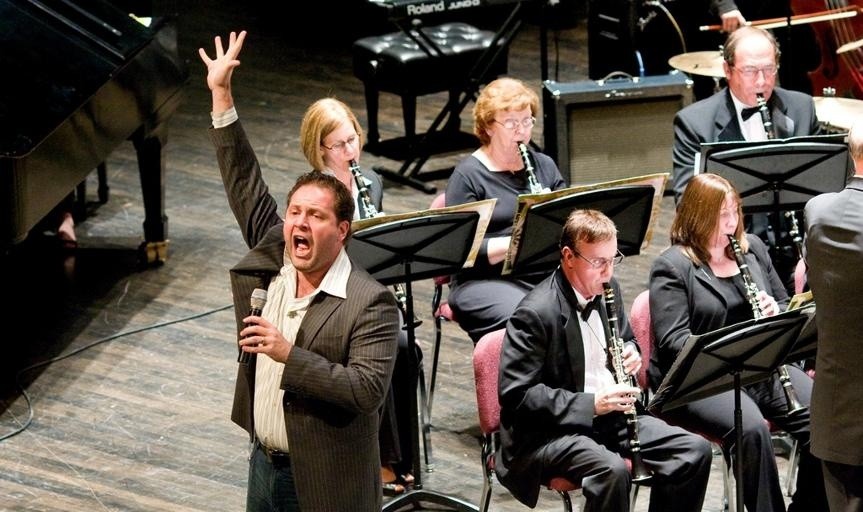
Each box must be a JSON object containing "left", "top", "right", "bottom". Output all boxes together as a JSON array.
[{"left": 1, "top": 1, "right": 197, "bottom": 268}]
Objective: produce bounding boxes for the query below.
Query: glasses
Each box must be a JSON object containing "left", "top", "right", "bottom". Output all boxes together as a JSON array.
[
  {"left": 574, "top": 249, "right": 624, "bottom": 273},
  {"left": 321, "top": 132, "right": 360, "bottom": 152},
  {"left": 494, "top": 116, "right": 536, "bottom": 130},
  {"left": 733, "top": 62, "right": 780, "bottom": 77}
]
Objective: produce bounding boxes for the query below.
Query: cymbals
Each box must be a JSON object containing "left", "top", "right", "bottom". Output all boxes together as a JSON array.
[{"left": 669, "top": 51, "right": 727, "bottom": 79}]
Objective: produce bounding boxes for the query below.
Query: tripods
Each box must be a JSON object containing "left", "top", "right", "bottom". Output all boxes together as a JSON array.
[{"left": 381, "top": 262, "right": 481, "bottom": 512}]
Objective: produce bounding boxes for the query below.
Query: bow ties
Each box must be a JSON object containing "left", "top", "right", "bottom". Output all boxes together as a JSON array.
[
  {"left": 581, "top": 295, "right": 601, "bottom": 321},
  {"left": 742, "top": 106, "right": 762, "bottom": 121}
]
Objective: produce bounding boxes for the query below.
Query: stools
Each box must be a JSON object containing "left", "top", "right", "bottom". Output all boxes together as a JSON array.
[{"left": 350, "top": 23, "right": 508, "bottom": 152}]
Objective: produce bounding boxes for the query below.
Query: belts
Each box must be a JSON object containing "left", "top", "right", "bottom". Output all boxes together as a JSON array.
[{"left": 256, "top": 438, "right": 289, "bottom": 464}]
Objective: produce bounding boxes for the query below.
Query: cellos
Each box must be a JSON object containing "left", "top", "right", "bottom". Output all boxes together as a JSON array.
[{"left": 791, "top": 0, "right": 863, "bottom": 99}]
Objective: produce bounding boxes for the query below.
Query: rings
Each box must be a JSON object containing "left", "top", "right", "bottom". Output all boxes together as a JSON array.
[{"left": 259, "top": 336, "right": 264, "bottom": 343}]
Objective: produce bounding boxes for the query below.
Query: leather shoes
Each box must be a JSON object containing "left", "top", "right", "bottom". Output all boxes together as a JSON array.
[{"left": 382, "top": 472, "right": 415, "bottom": 498}]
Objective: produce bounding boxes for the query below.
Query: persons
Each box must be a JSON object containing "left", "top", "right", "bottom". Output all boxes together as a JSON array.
[
  {"left": 441, "top": 73, "right": 568, "bottom": 348},
  {"left": 490, "top": 208, "right": 711, "bottom": 512},
  {"left": 673, "top": 24, "right": 817, "bottom": 297},
  {"left": 647, "top": 172, "right": 827, "bottom": 512},
  {"left": 711, "top": 0, "right": 744, "bottom": 34},
  {"left": 801, "top": 116, "right": 862, "bottom": 512},
  {"left": 298, "top": 89, "right": 424, "bottom": 498},
  {"left": 198, "top": 27, "right": 400, "bottom": 512}
]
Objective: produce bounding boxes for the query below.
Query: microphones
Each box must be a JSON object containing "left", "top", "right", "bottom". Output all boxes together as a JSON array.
[{"left": 238, "top": 287, "right": 268, "bottom": 364}]
